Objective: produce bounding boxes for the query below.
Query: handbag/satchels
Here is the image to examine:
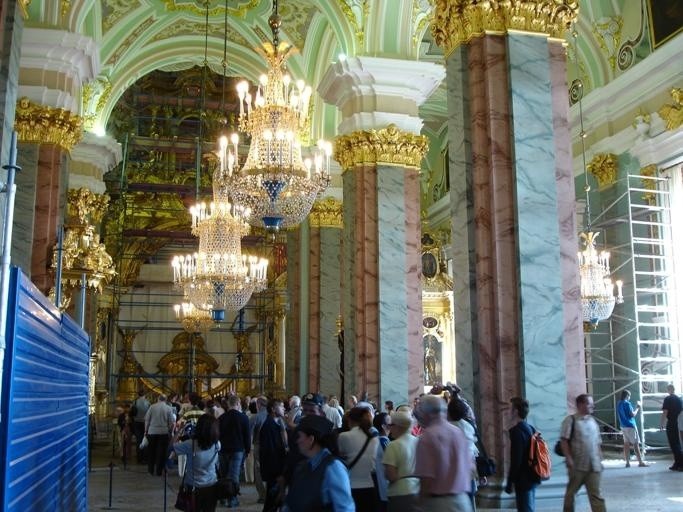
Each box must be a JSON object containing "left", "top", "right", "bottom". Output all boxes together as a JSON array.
[
  {"left": 175, "top": 492, "right": 197, "bottom": 512},
  {"left": 128, "top": 406, "right": 137, "bottom": 417},
  {"left": 475, "top": 458, "right": 496, "bottom": 476},
  {"left": 217, "top": 477, "right": 239, "bottom": 499},
  {"left": 555, "top": 440, "right": 571, "bottom": 456}
]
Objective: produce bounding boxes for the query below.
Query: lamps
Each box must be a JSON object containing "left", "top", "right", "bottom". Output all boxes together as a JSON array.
[
  {"left": 170, "top": 0, "right": 269, "bottom": 320},
  {"left": 156, "top": 0, "right": 221, "bottom": 333},
  {"left": 575, "top": 42, "right": 625, "bottom": 330},
  {"left": 217, "top": 1, "right": 334, "bottom": 243}
]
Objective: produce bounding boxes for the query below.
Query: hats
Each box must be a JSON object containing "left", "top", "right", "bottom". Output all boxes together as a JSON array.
[
  {"left": 299, "top": 414, "right": 333, "bottom": 435},
  {"left": 302, "top": 392, "right": 324, "bottom": 406}
]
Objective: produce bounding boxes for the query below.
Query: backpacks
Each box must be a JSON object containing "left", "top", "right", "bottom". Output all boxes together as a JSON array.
[{"left": 515, "top": 425, "right": 551, "bottom": 479}]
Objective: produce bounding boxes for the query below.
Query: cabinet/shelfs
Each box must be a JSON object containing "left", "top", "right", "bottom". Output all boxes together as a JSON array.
[
  {"left": 108, "top": 116, "right": 272, "bottom": 414},
  {"left": 577, "top": 174, "right": 683, "bottom": 459}
]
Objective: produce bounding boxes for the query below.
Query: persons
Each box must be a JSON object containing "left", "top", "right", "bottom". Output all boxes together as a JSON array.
[
  {"left": 660, "top": 384, "right": 683, "bottom": 471},
  {"left": 560, "top": 394, "right": 607, "bottom": 512},
  {"left": 118, "top": 382, "right": 479, "bottom": 512},
  {"left": 616, "top": 389, "right": 651, "bottom": 467},
  {"left": 505, "top": 397, "right": 541, "bottom": 512}
]
